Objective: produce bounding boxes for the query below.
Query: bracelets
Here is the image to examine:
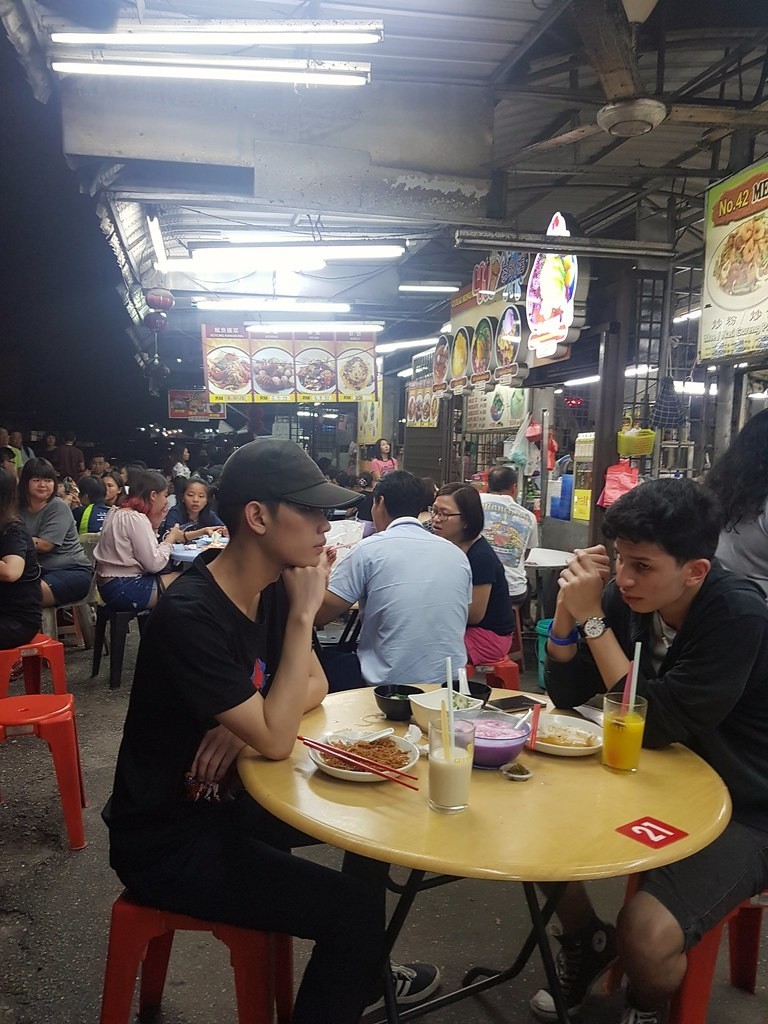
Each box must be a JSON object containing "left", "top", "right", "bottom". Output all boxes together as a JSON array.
[
  {"left": 184, "top": 531, "right": 192, "bottom": 542},
  {"left": 547, "top": 621, "right": 577, "bottom": 646}
]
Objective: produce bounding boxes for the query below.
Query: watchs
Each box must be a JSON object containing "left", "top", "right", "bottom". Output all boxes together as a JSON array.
[{"left": 577, "top": 616, "right": 611, "bottom": 639}]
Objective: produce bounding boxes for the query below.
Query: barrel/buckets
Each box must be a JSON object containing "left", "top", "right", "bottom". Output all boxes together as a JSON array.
[{"left": 535, "top": 619, "right": 580, "bottom": 689}]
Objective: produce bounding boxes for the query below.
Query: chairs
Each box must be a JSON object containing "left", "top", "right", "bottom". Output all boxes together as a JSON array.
[{"left": 40, "top": 532, "right": 101, "bottom": 652}]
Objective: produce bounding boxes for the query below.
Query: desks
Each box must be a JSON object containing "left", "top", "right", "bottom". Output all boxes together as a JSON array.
[{"left": 523, "top": 548, "right": 576, "bottom": 633}]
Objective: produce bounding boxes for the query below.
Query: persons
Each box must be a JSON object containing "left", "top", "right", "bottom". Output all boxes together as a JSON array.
[
  {"left": 9, "top": 457, "right": 94, "bottom": 680},
  {"left": 479, "top": 467, "right": 539, "bottom": 631},
  {"left": 527, "top": 478, "right": 768, "bottom": 1024},
  {"left": 0, "top": 467, "right": 42, "bottom": 651},
  {"left": 94, "top": 472, "right": 183, "bottom": 610},
  {"left": 120, "top": 444, "right": 223, "bottom": 513},
  {"left": 314, "top": 470, "right": 473, "bottom": 695},
  {"left": 702, "top": 408, "right": 768, "bottom": 602},
  {"left": 429, "top": 482, "right": 513, "bottom": 666},
  {"left": 318, "top": 457, "right": 377, "bottom": 538},
  {"left": 371, "top": 438, "right": 399, "bottom": 481},
  {"left": 0, "top": 428, "right": 128, "bottom": 535},
  {"left": 101, "top": 440, "right": 439, "bottom": 1024},
  {"left": 165, "top": 478, "right": 228, "bottom": 544}
]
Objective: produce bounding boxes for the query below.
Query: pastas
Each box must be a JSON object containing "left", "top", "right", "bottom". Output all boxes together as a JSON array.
[{"left": 321, "top": 736, "right": 409, "bottom": 773}]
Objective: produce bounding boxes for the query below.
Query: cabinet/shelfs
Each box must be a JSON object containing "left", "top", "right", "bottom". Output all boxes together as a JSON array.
[{"left": 571, "top": 454, "right": 592, "bottom": 526}]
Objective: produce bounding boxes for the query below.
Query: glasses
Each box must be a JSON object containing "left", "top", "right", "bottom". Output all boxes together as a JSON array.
[{"left": 428, "top": 506, "right": 461, "bottom": 521}]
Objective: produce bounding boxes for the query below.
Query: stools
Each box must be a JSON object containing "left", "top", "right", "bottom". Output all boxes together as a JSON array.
[{"left": 0, "top": 597, "right": 768, "bottom": 1024}]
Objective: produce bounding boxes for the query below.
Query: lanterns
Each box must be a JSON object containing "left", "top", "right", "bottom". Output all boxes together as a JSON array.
[
  {"left": 145, "top": 312, "right": 168, "bottom": 332},
  {"left": 146, "top": 289, "right": 174, "bottom": 312}
]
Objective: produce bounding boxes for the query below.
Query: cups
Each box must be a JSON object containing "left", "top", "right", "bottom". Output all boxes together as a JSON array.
[
  {"left": 428, "top": 718, "right": 477, "bottom": 814},
  {"left": 602, "top": 693, "right": 648, "bottom": 775}
]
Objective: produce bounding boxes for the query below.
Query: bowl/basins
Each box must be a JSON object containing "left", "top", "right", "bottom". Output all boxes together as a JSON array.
[
  {"left": 407, "top": 687, "right": 484, "bottom": 733},
  {"left": 447, "top": 710, "right": 532, "bottom": 767},
  {"left": 374, "top": 684, "right": 425, "bottom": 720},
  {"left": 442, "top": 680, "right": 492, "bottom": 708}
]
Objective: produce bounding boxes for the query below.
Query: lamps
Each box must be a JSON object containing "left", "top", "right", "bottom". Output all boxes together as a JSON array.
[
  {"left": 46, "top": 51, "right": 371, "bottom": 85},
  {"left": 39, "top": 14, "right": 385, "bottom": 45},
  {"left": 454, "top": 231, "right": 676, "bottom": 259}
]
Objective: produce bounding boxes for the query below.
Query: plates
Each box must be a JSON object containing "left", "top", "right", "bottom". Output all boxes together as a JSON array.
[
  {"left": 407, "top": 391, "right": 440, "bottom": 421},
  {"left": 296, "top": 347, "right": 336, "bottom": 394},
  {"left": 513, "top": 713, "right": 604, "bottom": 755},
  {"left": 206, "top": 346, "right": 251, "bottom": 394},
  {"left": 308, "top": 732, "right": 419, "bottom": 781},
  {"left": 337, "top": 348, "right": 376, "bottom": 394},
  {"left": 707, "top": 216, "right": 768, "bottom": 310},
  {"left": 252, "top": 347, "right": 295, "bottom": 394}
]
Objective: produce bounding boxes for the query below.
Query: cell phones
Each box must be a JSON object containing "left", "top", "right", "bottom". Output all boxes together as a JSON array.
[
  {"left": 179, "top": 520, "right": 197, "bottom": 530},
  {"left": 488, "top": 695, "right": 546, "bottom": 713}
]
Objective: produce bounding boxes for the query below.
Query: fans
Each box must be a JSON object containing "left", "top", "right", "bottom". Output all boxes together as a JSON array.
[{"left": 478, "top": 0, "right": 768, "bottom": 171}]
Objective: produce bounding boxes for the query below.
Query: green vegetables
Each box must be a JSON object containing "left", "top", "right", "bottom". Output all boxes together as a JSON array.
[{"left": 713, "top": 213, "right": 768, "bottom": 295}]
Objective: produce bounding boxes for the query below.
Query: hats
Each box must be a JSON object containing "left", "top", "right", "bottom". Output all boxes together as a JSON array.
[{"left": 214, "top": 437, "right": 366, "bottom": 510}]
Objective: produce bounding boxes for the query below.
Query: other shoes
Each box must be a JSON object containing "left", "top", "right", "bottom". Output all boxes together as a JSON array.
[
  {"left": 10, "top": 661, "right": 24, "bottom": 680},
  {"left": 523, "top": 617, "right": 535, "bottom": 631}
]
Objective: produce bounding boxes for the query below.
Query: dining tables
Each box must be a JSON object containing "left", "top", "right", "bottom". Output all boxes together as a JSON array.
[
  {"left": 236, "top": 684, "right": 732, "bottom": 1024},
  {"left": 166, "top": 535, "right": 230, "bottom": 561}
]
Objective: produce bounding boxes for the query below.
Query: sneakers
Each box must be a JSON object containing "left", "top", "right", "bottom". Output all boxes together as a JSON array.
[
  {"left": 530, "top": 920, "right": 620, "bottom": 1017},
  {"left": 620, "top": 992, "right": 668, "bottom": 1024},
  {"left": 361, "top": 960, "right": 441, "bottom": 1015}
]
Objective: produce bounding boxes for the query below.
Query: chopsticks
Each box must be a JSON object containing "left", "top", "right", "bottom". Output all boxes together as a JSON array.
[{"left": 297, "top": 736, "right": 419, "bottom": 791}]
